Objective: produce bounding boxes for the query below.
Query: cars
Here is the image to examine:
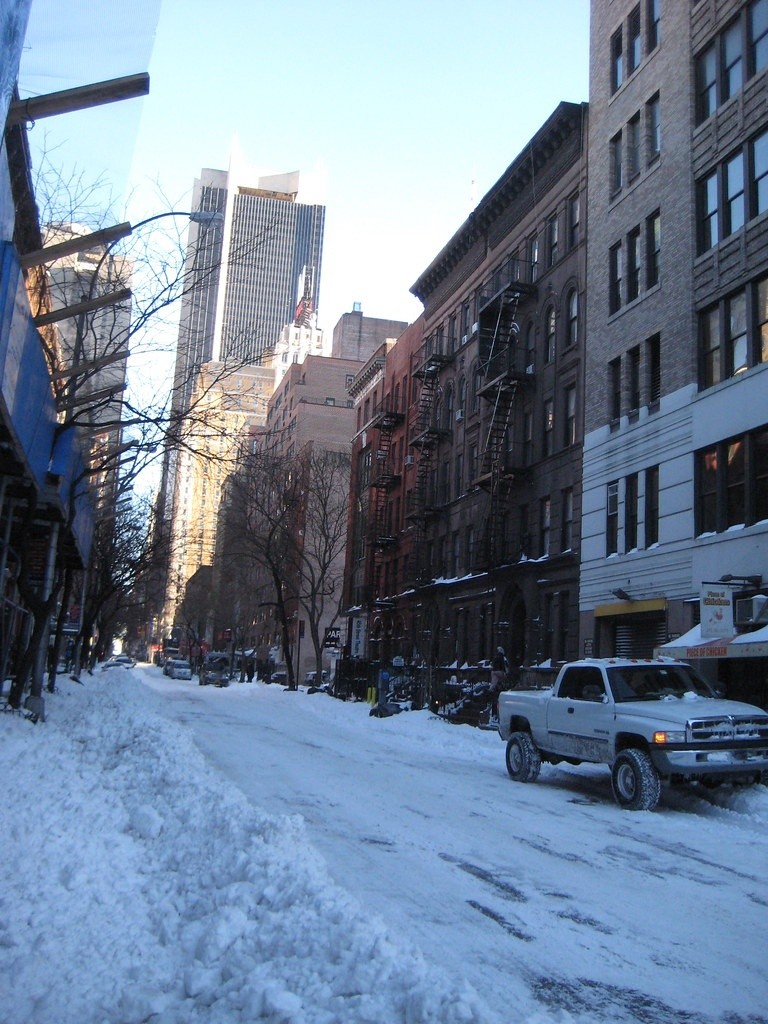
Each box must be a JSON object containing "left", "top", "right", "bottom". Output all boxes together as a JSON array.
[
  {"left": 163, "top": 660, "right": 191, "bottom": 681},
  {"left": 102, "top": 656, "right": 137, "bottom": 669}
]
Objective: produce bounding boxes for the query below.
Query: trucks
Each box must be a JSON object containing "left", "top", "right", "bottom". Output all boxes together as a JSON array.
[{"left": 198, "top": 652, "right": 231, "bottom": 687}]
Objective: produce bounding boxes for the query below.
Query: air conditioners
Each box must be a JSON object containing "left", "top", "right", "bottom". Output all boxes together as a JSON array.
[{"left": 735, "top": 597, "right": 768, "bottom": 624}]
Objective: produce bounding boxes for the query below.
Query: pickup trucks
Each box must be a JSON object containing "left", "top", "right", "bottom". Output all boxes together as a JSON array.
[{"left": 499, "top": 657, "right": 767, "bottom": 814}]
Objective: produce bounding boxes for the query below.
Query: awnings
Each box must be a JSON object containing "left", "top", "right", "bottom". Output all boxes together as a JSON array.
[{"left": 652, "top": 620, "right": 768, "bottom": 661}]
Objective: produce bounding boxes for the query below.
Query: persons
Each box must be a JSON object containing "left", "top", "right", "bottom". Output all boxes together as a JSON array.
[{"left": 489, "top": 653, "right": 507, "bottom": 723}]
